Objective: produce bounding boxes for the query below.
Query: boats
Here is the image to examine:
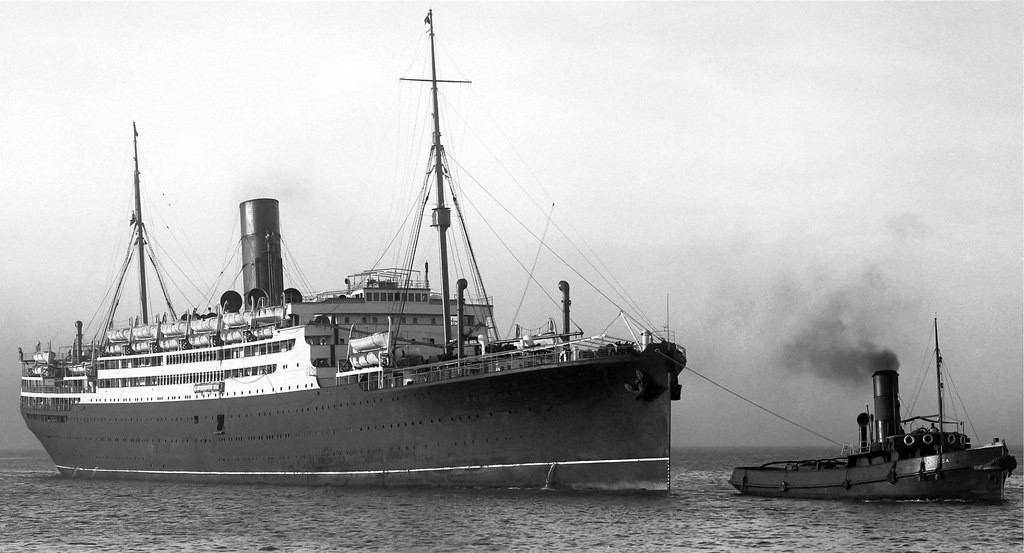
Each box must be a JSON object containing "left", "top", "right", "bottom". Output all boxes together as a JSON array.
[
  {"left": 726, "top": 308, "right": 1017, "bottom": 506},
  {"left": 17, "top": 0, "right": 688, "bottom": 494}
]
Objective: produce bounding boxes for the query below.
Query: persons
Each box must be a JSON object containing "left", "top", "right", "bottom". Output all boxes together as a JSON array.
[{"left": 595, "top": 339, "right": 634, "bottom": 356}]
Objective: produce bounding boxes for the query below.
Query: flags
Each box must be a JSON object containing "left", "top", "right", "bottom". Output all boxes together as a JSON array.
[{"left": 129, "top": 214, "right": 135, "bottom": 227}]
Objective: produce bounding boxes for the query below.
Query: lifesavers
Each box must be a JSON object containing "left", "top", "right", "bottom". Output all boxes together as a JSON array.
[
  {"left": 922, "top": 434, "right": 934, "bottom": 445},
  {"left": 903, "top": 434, "right": 915, "bottom": 446},
  {"left": 947, "top": 434, "right": 956, "bottom": 444},
  {"left": 960, "top": 435, "right": 966, "bottom": 446}
]
